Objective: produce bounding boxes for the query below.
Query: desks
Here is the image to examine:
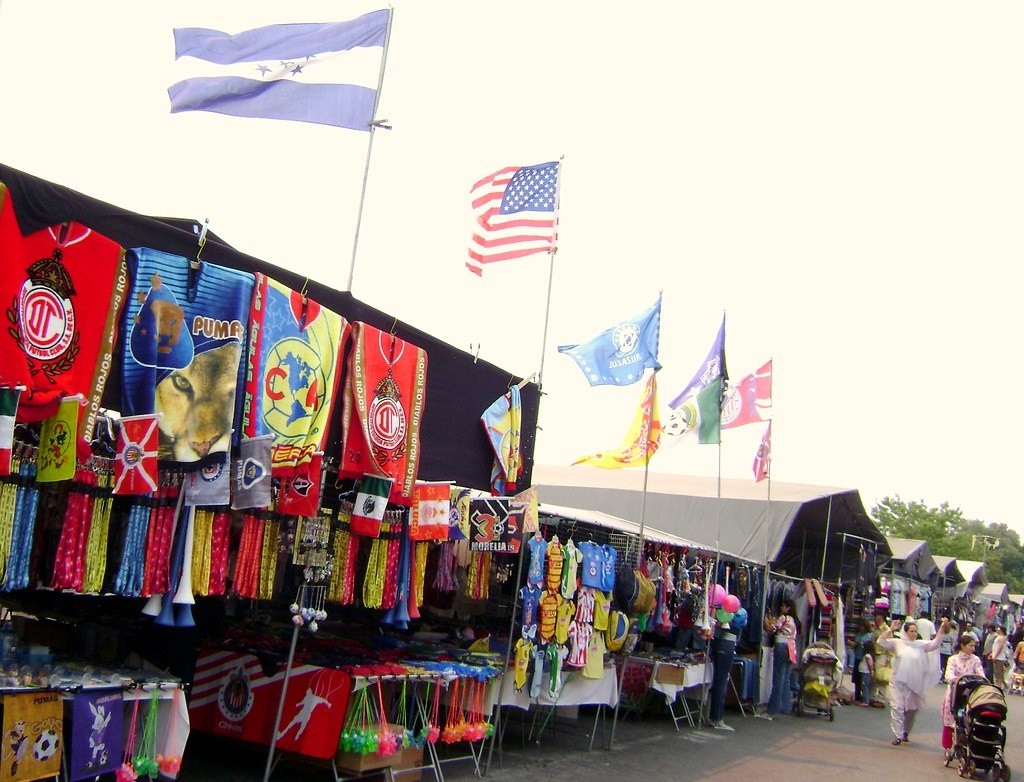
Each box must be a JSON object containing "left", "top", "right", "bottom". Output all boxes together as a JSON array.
[
  {"left": 190, "top": 650, "right": 502, "bottom": 782},
  {"left": 0, "top": 664, "right": 190, "bottom": 782},
  {"left": 407, "top": 661, "right": 618, "bottom": 766},
  {"left": 618, "top": 653, "right": 714, "bottom": 732},
  {"left": 729, "top": 660, "right": 757, "bottom": 716}
]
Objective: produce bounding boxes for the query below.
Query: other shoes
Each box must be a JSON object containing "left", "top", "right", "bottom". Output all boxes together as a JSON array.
[
  {"left": 855, "top": 701, "right": 869, "bottom": 708},
  {"left": 870, "top": 701, "right": 886, "bottom": 708},
  {"left": 902, "top": 733, "right": 909, "bottom": 742},
  {"left": 892, "top": 738, "right": 901, "bottom": 745}
]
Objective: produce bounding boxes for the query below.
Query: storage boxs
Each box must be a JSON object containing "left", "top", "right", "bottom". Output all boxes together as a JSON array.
[
  {"left": 386, "top": 744, "right": 424, "bottom": 782},
  {"left": 336, "top": 722, "right": 403, "bottom": 771}
]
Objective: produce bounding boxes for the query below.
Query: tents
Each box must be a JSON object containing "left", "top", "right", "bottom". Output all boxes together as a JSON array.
[
  {"left": 531, "top": 466, "right": 894, "bottom": 713},
  {"left": 885, "top": 537, "right": 1024, "bottom": 616},
  {"left": 0, "top": 163, "right": 547, "bottom": 782}
]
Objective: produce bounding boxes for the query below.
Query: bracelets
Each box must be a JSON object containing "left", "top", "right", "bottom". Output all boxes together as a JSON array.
[{"left": 888, "top": 628, "right": 891, "bottom": 633}]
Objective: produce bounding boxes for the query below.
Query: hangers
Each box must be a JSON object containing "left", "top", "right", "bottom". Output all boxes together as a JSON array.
[
  {"left": 338, "top": 488, "right": 404, "bottom": 514},
  {"left": 88, "top": 424, "right": 116, "bottom": 462},
  {"left": 859, "top": 539, "right": 866, "bottom": 561},
  {"left": 13, "top": 423, "right": 38, "bottom": 450}
]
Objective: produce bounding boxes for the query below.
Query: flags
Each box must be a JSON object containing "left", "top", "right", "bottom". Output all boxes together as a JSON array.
[
  {"left": 469, "top": 497, "right": 526, "bottom": 553},
  {"left": 278, "top": 452, "right": 321, "bottom": 517},
  {"left": 409, "top": 482, "right": 450, "bottom": 540},
  {"left": 167, "top": 8, "right": 393, "bottom": 132},
  {"left": 465, "top": 161, "right": 562, "bottom": 277},
  {"left": 0, "top": 386, "right": 21, "bottom": 476},
  {"left": 230, "top": 434, "right": 271, "bottom": 510},
  {"left": 570, "top": 368, "right": 670, "bottom": 468},
  {"left": 111, "top": 414, "right": 159, "bottom": 495},
  {"left": 720, "top": 360, "right": 773, "bottom": 430},
  {"left": 660, "top": 314, "right": 729, "bottom": 444},
  {"left": 36, "top": 395, "right": 79, "bottom": 482},
  {"left": 555, "top": 289, "right": 666, "bottom": 390},
  {"left": 752, "top": 419, "right": 772, "bottom": 483},
  {"left": 349, "top": 472, "right": 392, "bottom": 538},
  {"left": 185, "top": 433, "right": 231, "bottom": 506}
]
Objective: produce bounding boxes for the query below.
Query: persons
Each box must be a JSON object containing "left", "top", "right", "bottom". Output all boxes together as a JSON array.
[
  {"left": 876, "top": 617, "right": 949, "bottom": 745},
  {"left": 709, "top": 631, "right": 737, "bottom": 731},
  {"left": 848, "top": 609, "right": 1024, "bottom": 708},
  {"left": 764, "top": 599, "right": 802, "bottom": 715},
  {"left": 942, "top": 635, "right": 986, "bottom": 749}
]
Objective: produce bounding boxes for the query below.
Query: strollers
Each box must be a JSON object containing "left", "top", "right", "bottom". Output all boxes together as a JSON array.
[
  {"left": 944, "top": 674, "right": 1011, "bottom": 782},
  {"left": 792, "top": 642, "right": 838, "bottom": 721}
]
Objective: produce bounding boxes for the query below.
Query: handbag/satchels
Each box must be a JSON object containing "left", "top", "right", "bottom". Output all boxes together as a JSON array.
[{"left": 618, "top": 564, "right": 657, "bottom": 614}]
